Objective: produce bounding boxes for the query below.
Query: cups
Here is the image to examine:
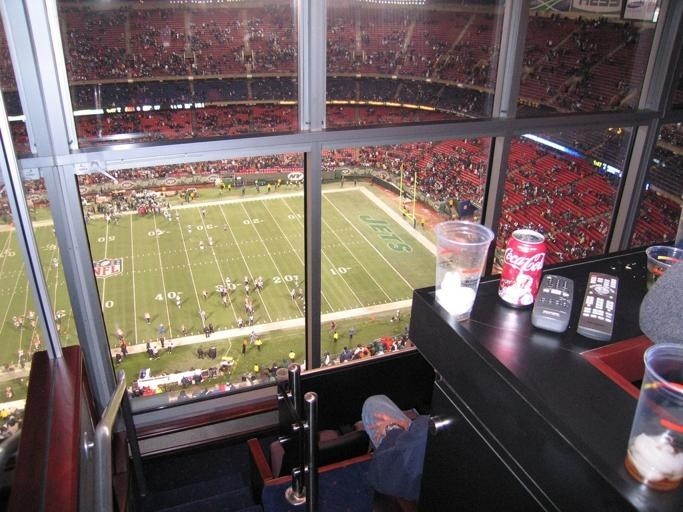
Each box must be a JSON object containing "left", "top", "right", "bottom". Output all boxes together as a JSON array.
[
  {"left": 621, "top": 341, "right": 682, "bottom": 493},
  {"left": 643, "top": 245, "right": 682, "bottom": 292},
  {"left": 430, "top": 219, "right": 497, "bottom": 325}
]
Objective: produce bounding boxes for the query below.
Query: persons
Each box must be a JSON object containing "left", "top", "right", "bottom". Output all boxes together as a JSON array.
[
  {"left": 362, "top": 260, "right": 683, "bottom": 502},
  {"left": 1, "top": 276, "right": 415, "bottom": 452},
  {"left": 22, "top": 178, "right": 50, "bottom": 220},
  {"left": 57, "top": 0, "right": 683, "bottom": 263},
  {"left": 1, "top": 184, "right": 15, "bottom": 227},
  {"left": 1, "top": 17, "right": 31, "bottom": 154}
]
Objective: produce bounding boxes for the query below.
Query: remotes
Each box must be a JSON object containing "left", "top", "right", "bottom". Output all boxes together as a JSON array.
[
  {"left": 531, "top": 274, "right": 574, "bottom": 333},
  {"left": 576, "top": 271, "right": 620, "bottom": 341}
]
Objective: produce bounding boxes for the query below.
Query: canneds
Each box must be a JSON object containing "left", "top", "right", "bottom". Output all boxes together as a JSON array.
[{"left": 498, "top": 229, "right": 546, "bottom": 308}]
